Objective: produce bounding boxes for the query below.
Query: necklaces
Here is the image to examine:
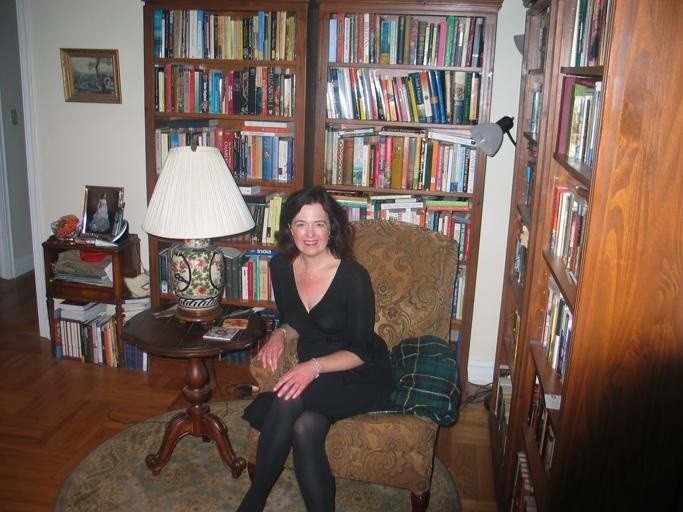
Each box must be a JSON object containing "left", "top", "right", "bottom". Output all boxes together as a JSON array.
[{"left": 300, "top": 248, "right": 331, "bottom": 280}]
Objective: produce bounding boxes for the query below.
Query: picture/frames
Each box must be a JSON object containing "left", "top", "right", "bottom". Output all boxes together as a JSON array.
[
  {"left": 79, "top": 184, "right": 124, "bottom": 239},
  {"left": 54, "top": 46, "right": 124, "bottom": 108}
]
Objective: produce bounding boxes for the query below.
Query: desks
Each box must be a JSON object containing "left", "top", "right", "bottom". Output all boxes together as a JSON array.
[{"left": 122, "top": 302, "right": 265, "bottom": 482}]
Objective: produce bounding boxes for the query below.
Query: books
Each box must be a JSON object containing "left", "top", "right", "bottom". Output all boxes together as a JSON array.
[{"left": 50, "top": 298, "right": 151, "bottom": 372}]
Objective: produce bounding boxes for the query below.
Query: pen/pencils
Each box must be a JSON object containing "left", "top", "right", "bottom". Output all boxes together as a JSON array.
[{"left": 222, "top": 309, "right": 252, "bottom": 319}]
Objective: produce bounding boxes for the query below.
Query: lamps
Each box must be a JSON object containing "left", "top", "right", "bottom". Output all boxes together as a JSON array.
[
  {"left": 141, "top": 122, "right": 255, "bottom": 328},
  {"left": 469, "top": 117, "right": 519, "bottom": 161}
]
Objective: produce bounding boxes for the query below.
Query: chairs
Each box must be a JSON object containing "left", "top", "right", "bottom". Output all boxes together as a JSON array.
[{"left": 245, "top": 216, "right": 468, "bottom": 509}]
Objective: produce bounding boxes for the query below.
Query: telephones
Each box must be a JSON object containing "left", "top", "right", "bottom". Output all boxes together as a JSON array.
[{"left": 102, "top": 202, "right": 129, "bottom": 244}]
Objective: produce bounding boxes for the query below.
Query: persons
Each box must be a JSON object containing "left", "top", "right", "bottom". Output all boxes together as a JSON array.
[
  {"left": 87, "top": 193, "right": 110, "bottom": 233},
  {"left": 234, "top": 186, "right": 394, "bottom": 512}
]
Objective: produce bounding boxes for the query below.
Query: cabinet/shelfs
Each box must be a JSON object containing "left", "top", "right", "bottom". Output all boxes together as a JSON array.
[
  {"left": 38, "top": 222, "right": 142, "bottom": 368},
  {"left": 141, "top": 1, "right": 313, "bottom": 371},
  {"left": 485, "top": 2, "right": 552, "bottom": 512},
  {"left": 503, "top": 1, "right": 682, "bottom": 510},
  {"left": 299, "top": 1, "right": 502, "bottom": 408}
]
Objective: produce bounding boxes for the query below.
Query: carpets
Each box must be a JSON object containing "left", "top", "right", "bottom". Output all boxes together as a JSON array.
[{"left": 51, "top": 396, "right": 472, "bottom": 509}]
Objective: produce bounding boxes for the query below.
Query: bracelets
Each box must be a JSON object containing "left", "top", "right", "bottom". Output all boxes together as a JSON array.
[{"left": 311, "top": 357, "right": 321, "bottom": 379}]
